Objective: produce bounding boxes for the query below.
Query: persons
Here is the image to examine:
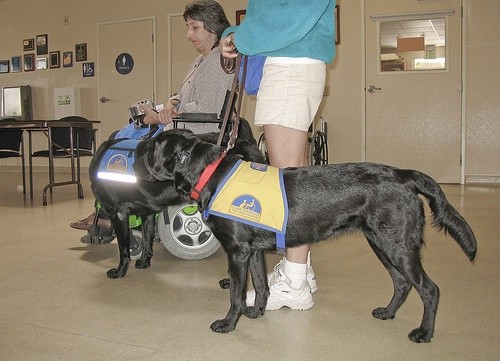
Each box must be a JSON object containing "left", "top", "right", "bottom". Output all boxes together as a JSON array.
[
  {"left": 219, "top": 0, "right": 336, "bottom": 310},
  {"left": 69, "top": 0, "right": 234, "bottom": 236}
]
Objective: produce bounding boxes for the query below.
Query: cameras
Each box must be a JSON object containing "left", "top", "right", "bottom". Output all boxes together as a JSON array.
[{"left": 129, "top": 100, "right": 153, "bottom": 116}]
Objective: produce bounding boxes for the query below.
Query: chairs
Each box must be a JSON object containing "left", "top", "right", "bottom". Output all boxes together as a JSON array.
[
  {"left": 0, "top": 118, "right": 25, "bottom": 194},
  {"left": 33, "top": 116, "right": 98, "bottom": 199}
]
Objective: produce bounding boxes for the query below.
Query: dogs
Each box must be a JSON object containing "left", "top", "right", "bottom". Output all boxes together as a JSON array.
[
  {"left": 88, "top": 132, "right": 267, "bottom": 289},
  {"left": 132, "top": 129, "right": 478, "bottom": 344}
]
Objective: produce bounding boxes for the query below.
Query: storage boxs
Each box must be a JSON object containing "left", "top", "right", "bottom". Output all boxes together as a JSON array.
[{"left": 54, "top": 86, "right": 82, "bottom": 120}]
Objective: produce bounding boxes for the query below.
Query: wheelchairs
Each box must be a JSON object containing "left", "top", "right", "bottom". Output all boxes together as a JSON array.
[
  {"left": 257, "top": 112, "right": 329, "bottom": 167},
  {"left": 77, "top": 87, "right": 242, "bottom": 261}
]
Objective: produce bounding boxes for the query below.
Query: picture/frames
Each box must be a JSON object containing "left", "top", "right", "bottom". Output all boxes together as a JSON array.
[
  {"left": 63, "top": 52, "right": 73, "bottom": 67},
  {"left": 23, "top": 39, "right": 34, "bottom": 51},
  {"left": 75, "top": 43, "right": 87, "bottom": 61},
  {"left": 24, "top": 53, "right": 35, "bottom": 71},
  {"left": 36, "top": 34, "right": 48, "bottom": 55},
  {"left": 11, "top": 55, "right": 22, "bottom": 73},
  {"left": 0, "top": 60, "right": 9, "bottom": 73},
  {"left": 36, "top": 58, "right": 48, "bottom": 70},
  {"left": 49, "top": 51, "right": 60, "bottom": 68}
]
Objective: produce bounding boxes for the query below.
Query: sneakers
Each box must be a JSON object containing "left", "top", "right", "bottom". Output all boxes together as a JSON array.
[
  {"left": 245, "top": 267, "right": 315, "bottom": 311},
  {"left": 267, "top": 255, "right": 318, "bottom": 294}
]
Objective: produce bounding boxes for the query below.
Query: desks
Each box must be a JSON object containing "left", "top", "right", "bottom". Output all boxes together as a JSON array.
[{"left": 0, "top": 121, "right": 101, "bottom": 205}]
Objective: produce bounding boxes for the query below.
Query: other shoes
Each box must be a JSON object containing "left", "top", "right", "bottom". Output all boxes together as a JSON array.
[
  {"left": 70, "top": 212, "right": 114, "bottom": 237},
  {"left": 80, "top": 229, "right": 117, "bottom": 245}
]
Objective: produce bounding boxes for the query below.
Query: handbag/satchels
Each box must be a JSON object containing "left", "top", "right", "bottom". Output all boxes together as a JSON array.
[{"left": 237, "top": 55, "right": 267, "bottom": 96}]
